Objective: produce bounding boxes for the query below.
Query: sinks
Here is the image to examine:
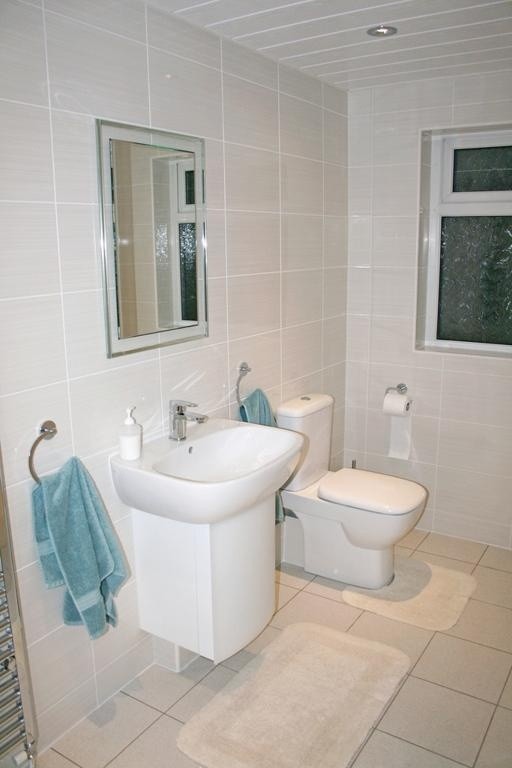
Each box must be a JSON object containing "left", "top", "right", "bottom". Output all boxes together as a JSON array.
[{"left": 111, "top": 418, "right": 304, "bottom": 524}]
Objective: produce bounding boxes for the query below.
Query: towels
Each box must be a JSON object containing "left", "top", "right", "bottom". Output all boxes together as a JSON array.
[
  {"left": 240, "top": 388, "right": 286, "bottom": 525},
  {"left": 30, "top": 456, "right": 129, "bottom": 641}
]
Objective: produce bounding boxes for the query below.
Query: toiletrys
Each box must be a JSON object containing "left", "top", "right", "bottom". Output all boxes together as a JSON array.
[{"left": 119, "top": 406, "right": 143, "bottom": 458}]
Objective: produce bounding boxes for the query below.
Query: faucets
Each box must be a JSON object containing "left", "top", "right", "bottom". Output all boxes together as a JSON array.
[{"left": 169, "top": 401, "right": 207, "bottom": 441}]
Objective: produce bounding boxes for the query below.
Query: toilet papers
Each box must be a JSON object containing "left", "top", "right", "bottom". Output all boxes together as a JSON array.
[{"left": 383, "top": 394, "right": 412, "bottom": 460}]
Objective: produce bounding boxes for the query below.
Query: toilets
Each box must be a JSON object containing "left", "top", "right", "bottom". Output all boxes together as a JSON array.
[{"left": 275, "top": 392, "right": 427, "bottom": 591}]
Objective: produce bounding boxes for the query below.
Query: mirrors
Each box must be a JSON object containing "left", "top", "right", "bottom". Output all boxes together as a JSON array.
[{"left": 96, "top": 117, "right": 210, "bottom": 359}]
